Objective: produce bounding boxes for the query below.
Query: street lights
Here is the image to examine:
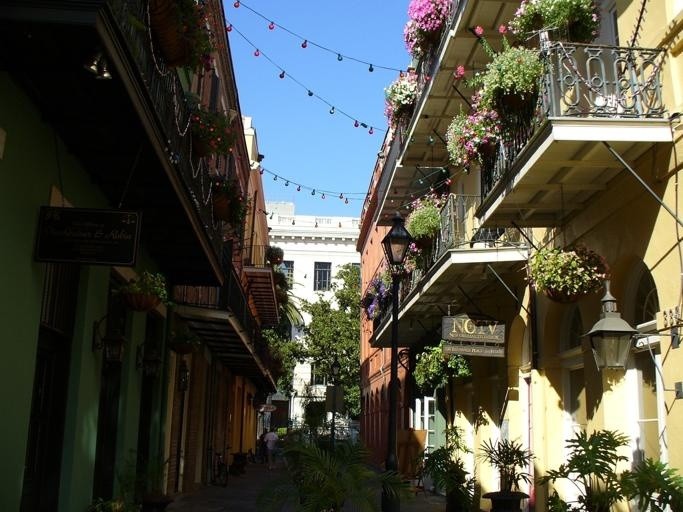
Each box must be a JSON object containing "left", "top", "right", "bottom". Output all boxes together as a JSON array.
[
  {"left": 382, "top": 210, "right": 413, "bottom": 511},
  {"left": 330, "top": 355, "right": 341, "bottom": 452}
]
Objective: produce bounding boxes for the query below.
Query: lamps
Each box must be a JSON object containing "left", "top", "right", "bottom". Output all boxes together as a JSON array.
[
  {"left": 580, "top": 278, "right": 681, "bottom": 372},
  {"left": 85, "top": 51, "right": 114, "bottom": 82}
]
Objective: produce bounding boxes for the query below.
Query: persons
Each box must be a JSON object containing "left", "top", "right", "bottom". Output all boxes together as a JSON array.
[{"left": 258, "top": 427, "right": 279, "bottom": 464}]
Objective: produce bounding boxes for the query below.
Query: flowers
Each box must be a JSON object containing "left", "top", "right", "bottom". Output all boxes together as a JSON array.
[
  {"left": 161, "top": 0, "right": 229, "bottom": 72},
  {"left": 522, "top": 243, "right": 611, "bottom": 299},
  {"left": 444, "top": 109, "right": 504, "bottom": 168},
  {"left": 360, "top": 254, "right": 412, "bottom": 321},
  {"left": 385, "top": 71, "right": 425, "bottom": 128},
  {"left": 187, "top": 90, "right": 238, "bottom": 160},
  {"left": 402, "top": 191, "right": 451, "bottom": 249},
  {"left": 508, "top": 0, "right": 600, "bottom": 46},
  {"left": 451, "top": 26, "right": 543, "bottom": 116},
  {"left": 405, "top": 0, "right": 452, "bottom": 59},
  {"left": 213, "top": 171, "right": 258, "bottom": 233}
]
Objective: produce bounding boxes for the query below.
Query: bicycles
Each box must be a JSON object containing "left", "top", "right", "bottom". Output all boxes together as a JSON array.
[{"left": 206, "top": 446, "right": 233, "bottom": 488}]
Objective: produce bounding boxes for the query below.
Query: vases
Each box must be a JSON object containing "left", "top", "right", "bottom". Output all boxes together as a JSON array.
[
  {"left": 425, "top": 22, "right": 443, "bottom": 46},
  {"left": 186, "top": 135, "right": 205, "bottom": 167},
  {"left": 402, "top": 93, "right": 418, "bottom": 118},
  {"left": 215, "top": 191, "right": 232, "bottom": 223},
  {"left": 545, "top": 287, "right": 586, "bottom": 304},
  {"left": 500, "top": 90, "right": 532, "bottom": 112},
  {"left": 525, "top": 11, "right": 555, "bottom": 47},
  {"left": 150, "top": 2, "right": 190, "bottom": 65},
  {"left": 416, "top": 232, "right": 435, "bottom": 248},
  {"left": 476, "top": 136, "right": 497, "bottom": 165}
]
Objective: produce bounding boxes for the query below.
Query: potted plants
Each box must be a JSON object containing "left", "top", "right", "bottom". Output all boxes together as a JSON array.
[
  {"left": 109, "top": 268, "right": 176, "bottom": 311},
  {"left": 115, "top": 429, "right": 188, "bottom": 512},
  {"left": 413, "top": 339, "right": 475, "bottom": 395},
  {"left": 167, "top": 327, "right": 201, "bottom": 355},
  {"left": 482, "top": 439, "right": 536, "bottom": 512}
]
